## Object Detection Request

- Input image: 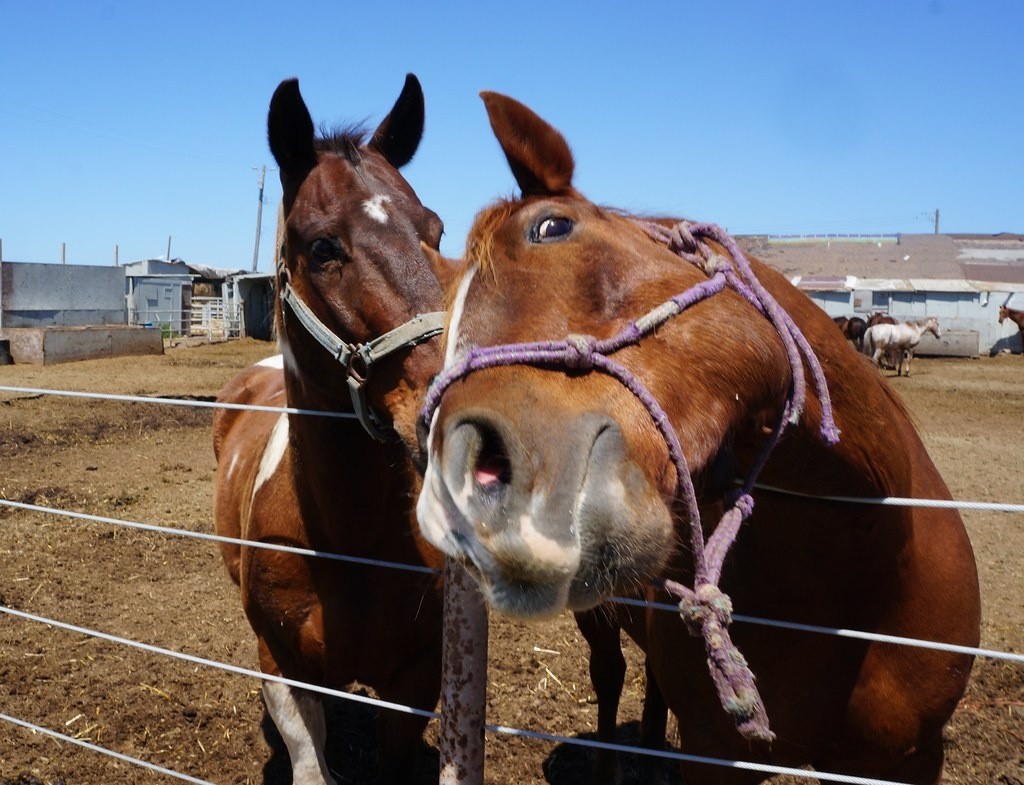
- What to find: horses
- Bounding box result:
[214,72,1023,785]
